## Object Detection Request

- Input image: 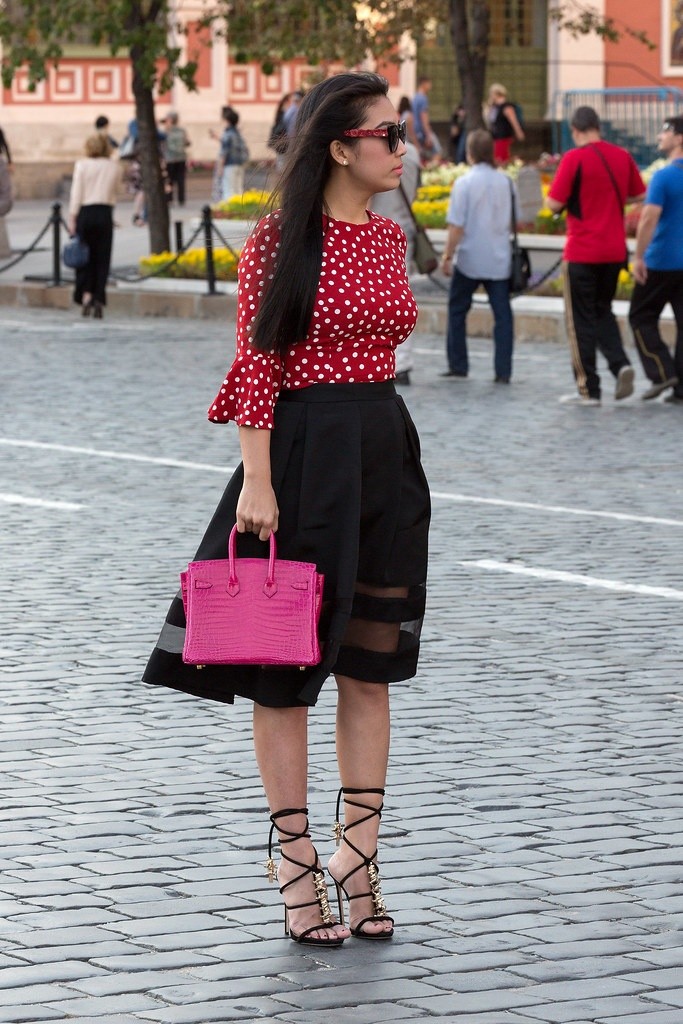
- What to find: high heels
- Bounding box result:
[329,783,395,940]
[267,807,351,946]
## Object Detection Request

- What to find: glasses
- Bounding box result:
[344,121,406,154]
[663,122,677,134]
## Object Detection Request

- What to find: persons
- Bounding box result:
[366,139,421,387]
[128,110,190,227]
[95,116,121,229]
[397,76,443,168]
[548,106,646,406]
[209,106,250,204]
[436,129,521,386]
[0,126,16,217]
[69,132,116,319]
[628,115,683,404]
[448,83,527,167]
[274,91,306,179]
[141,71,431,945]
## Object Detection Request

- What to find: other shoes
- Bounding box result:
[665,396,683,405]
[81,299,102,320]
[441,371,468,383]
[643,378,678,399]
[615,365,635,400]
[393,371,408,386]
[561,392,601,406]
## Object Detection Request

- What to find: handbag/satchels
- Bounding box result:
[62,233,89,268]
[506,245,531,292]
[180,520,323,671]
[413,229,437,273]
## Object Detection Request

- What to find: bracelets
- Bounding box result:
[442,254,453,260]
